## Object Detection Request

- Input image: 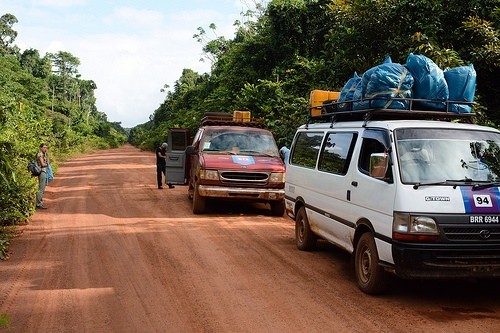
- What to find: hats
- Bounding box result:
[161,142,167,148]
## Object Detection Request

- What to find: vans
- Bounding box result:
[285,97,500,294]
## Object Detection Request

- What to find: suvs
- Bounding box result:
[165,116,286,216]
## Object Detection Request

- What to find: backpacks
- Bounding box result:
[46,163,54,186]
[28,153,41,175]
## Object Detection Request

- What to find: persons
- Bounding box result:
[36,143,49,209]
[155,143,175,189]
[278,143,290,166]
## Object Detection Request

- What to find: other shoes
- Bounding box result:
[38,206,47,209]
[157,186,162,188]
[169,185,175,188]
